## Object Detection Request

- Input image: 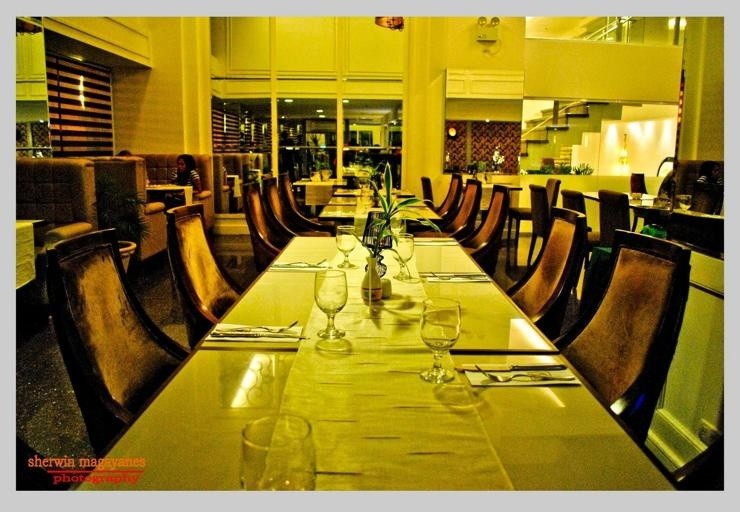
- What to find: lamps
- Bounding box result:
[476,15,499,42]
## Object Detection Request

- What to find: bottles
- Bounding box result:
[446,153,451,169]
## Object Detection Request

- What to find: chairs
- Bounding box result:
[15,144,722,490]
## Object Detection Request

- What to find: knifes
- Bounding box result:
[420,273,486,277]
[455,365,567,371]
[272,265,332,269]
[211,331,312,339]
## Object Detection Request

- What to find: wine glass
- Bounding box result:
[314,270,348,338]
[392,232,414,281]
[336,225,357,268]
[420,298,460,384]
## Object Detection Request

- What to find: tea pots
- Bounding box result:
[310,172,321,181]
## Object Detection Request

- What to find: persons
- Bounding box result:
[280,130,401,183]
[164,154,202,226]
[222,167,227,184]
[694,161,723,214]
[118,151,150,187]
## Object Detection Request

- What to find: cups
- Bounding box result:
[357,179,372,217]
[677,194,692,211]
[237,414,318,490]
[347,161,362,175]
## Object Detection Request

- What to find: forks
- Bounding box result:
[276,259,325,266]
[430,271,489,282]
[217,322,297,333]
[473,362,576,381]
